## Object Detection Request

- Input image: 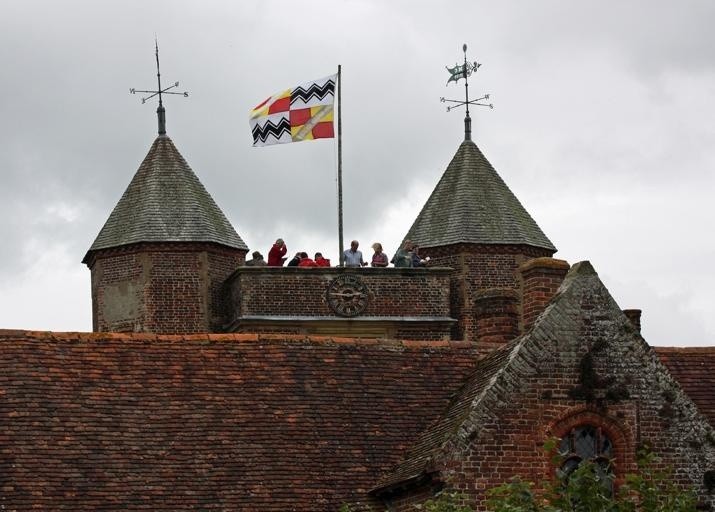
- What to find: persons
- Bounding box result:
[396,240,413,267]
[413,245,428,267]
[371,243,388,267]
[244,237,331,267]
[343,240,368,267]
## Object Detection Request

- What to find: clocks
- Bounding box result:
[327,276,369,318]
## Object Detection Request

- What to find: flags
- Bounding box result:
[248,72,338,147]
